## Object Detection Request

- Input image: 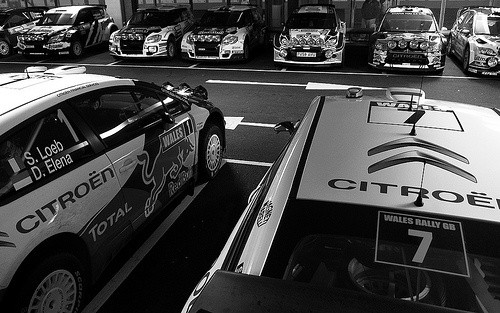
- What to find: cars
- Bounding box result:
[366,4,450,78]
[178,86,500,313]
[0,63,227,313]
[12,3,119,60]
[272,3,348,69]
[446,5,500,78]
[0,5,52,58]
[107,4,201,62]
[179,3,270,66]
